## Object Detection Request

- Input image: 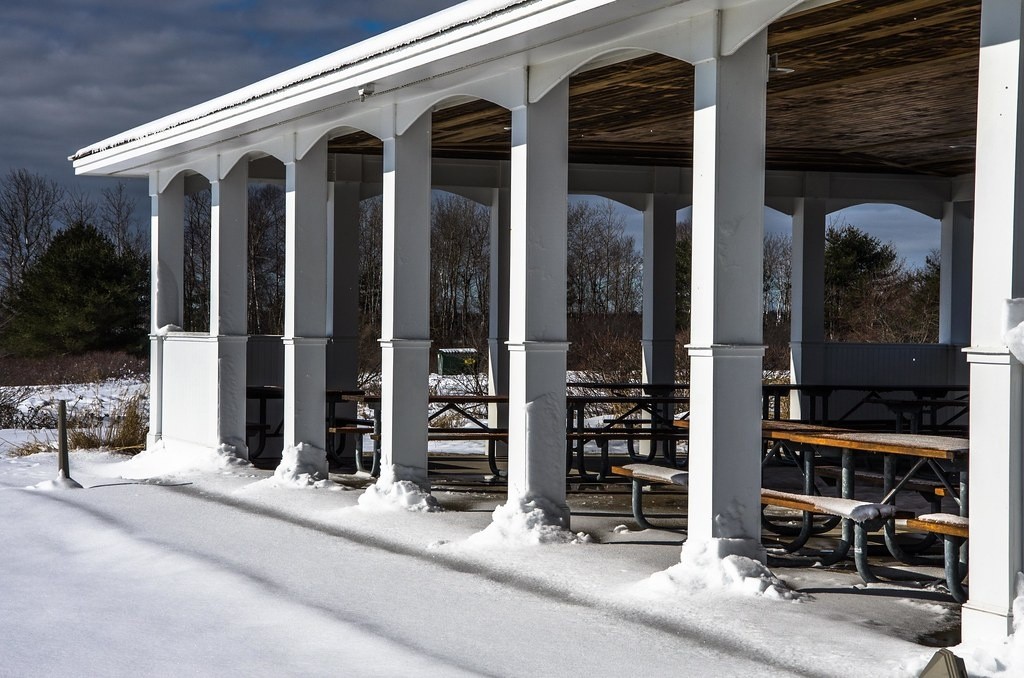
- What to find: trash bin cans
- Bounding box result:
[436,348,479,375]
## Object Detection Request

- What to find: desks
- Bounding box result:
[565,382,790,481]
[246,386,365,468]
[341,394,509,477]
[672,418,970,603]
[773,384,969,439]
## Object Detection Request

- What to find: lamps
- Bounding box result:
[767,52,795,83]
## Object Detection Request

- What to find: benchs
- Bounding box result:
[326,416,374,459]
[565,398,969,481]
[246,422,270,459]
[611,465,970,603]
[329,427,509,477]
